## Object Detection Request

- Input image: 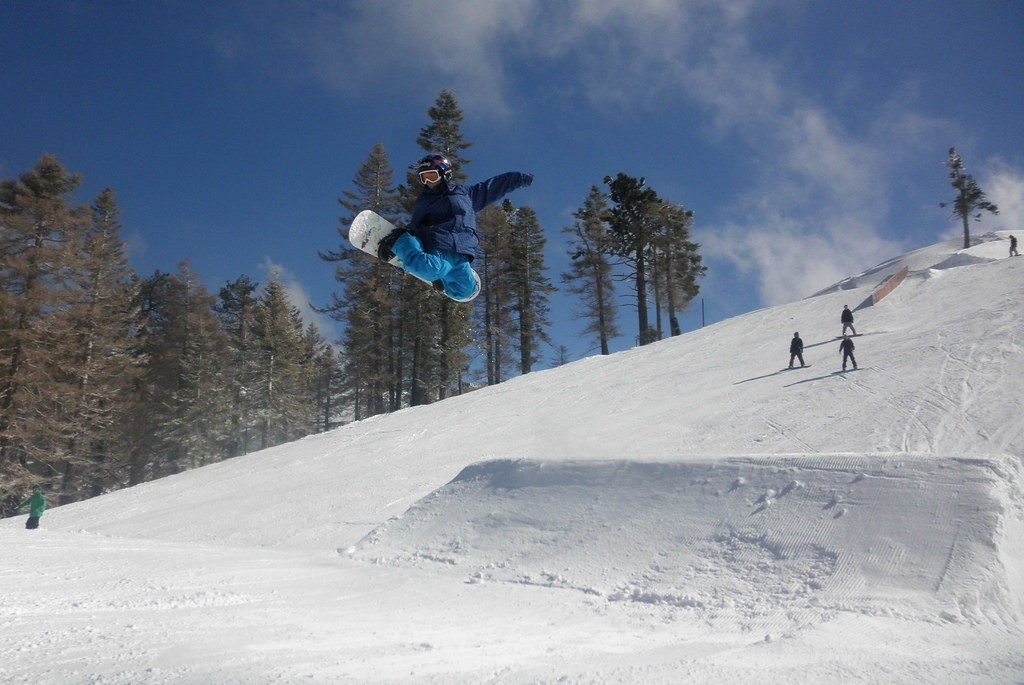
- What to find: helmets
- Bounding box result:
[416,154,452,182]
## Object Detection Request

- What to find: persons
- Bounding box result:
[14,484,45,529]
[841,305,856,336]
[378,155,534,303]
[1009,235,1018,256]
[789,332,805,367]
[839,333,858,371]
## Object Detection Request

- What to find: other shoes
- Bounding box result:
[432,280,444,292]
[378,229,409,264]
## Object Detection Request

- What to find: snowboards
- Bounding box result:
[348,209,482,303]
[778,364,813,373]
[830,367,865,375]
[834,333,864,340]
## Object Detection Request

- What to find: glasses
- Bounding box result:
[418,170,442,185]
